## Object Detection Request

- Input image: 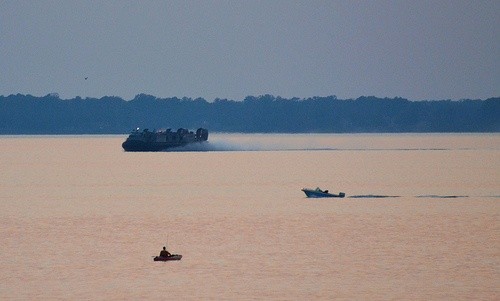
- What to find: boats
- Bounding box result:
[302,188,345,198]
[122,127,208,153]
[154,254,181,262]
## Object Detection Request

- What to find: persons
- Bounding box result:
[160,247,171,257]
